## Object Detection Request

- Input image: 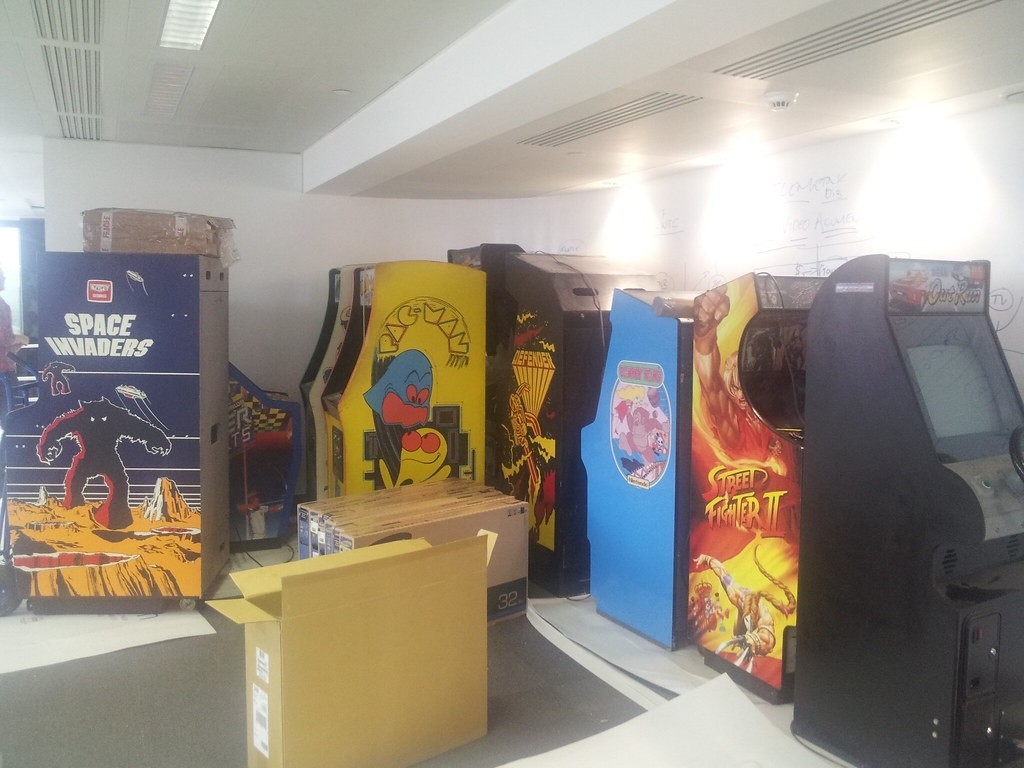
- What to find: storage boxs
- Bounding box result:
[296,476,531,630]
[201,528,499,767]
[83,207,238,256]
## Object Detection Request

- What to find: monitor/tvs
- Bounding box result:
[906,344,1003,437]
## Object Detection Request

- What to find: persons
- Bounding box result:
[0,264,29,432]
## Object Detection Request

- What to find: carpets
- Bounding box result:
[0,595,648,768]
